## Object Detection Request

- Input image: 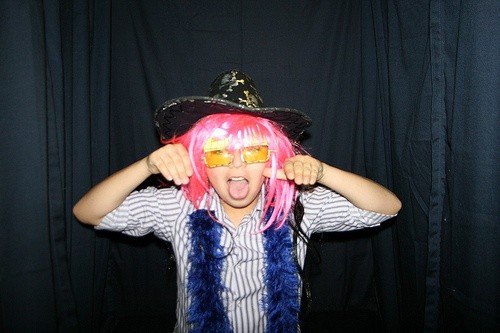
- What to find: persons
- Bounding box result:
[72,71,402,333]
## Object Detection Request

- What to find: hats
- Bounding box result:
[154,69,311,145]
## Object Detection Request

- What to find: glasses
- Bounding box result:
[203,144,270,168]
